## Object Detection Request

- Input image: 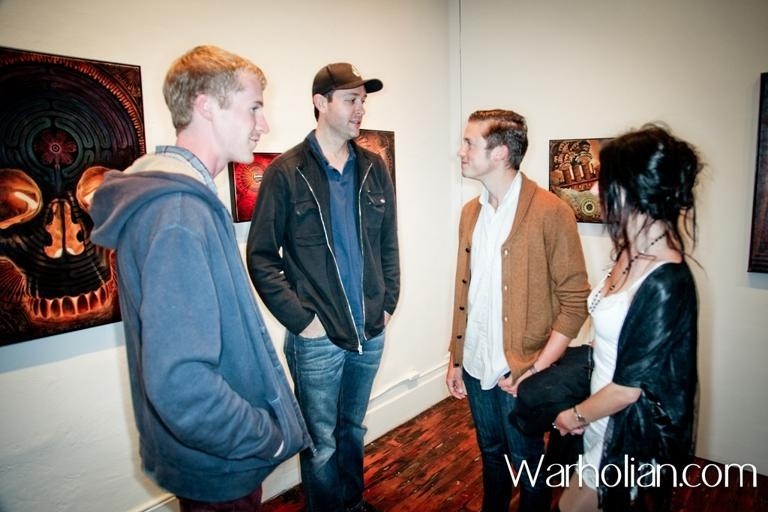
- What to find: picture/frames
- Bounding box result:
[355,128,397,197]
[745,71,768,275]
[0,43,148,350]
[226,151,283,224]
[547,136,611,225]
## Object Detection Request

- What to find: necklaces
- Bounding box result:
[588,230,666,315]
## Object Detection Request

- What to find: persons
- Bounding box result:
[246,62,400,512]
[550,123,703,512]
[444,110,592,512]
[88,45,317,512]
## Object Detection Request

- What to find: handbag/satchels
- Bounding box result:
[509,344,594,440]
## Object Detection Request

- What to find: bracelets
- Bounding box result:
[572,405,590,429]
[529,366,539,375]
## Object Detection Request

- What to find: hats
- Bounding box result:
[313,62,384,97]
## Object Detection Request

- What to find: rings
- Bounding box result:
[551,421,557,429]
